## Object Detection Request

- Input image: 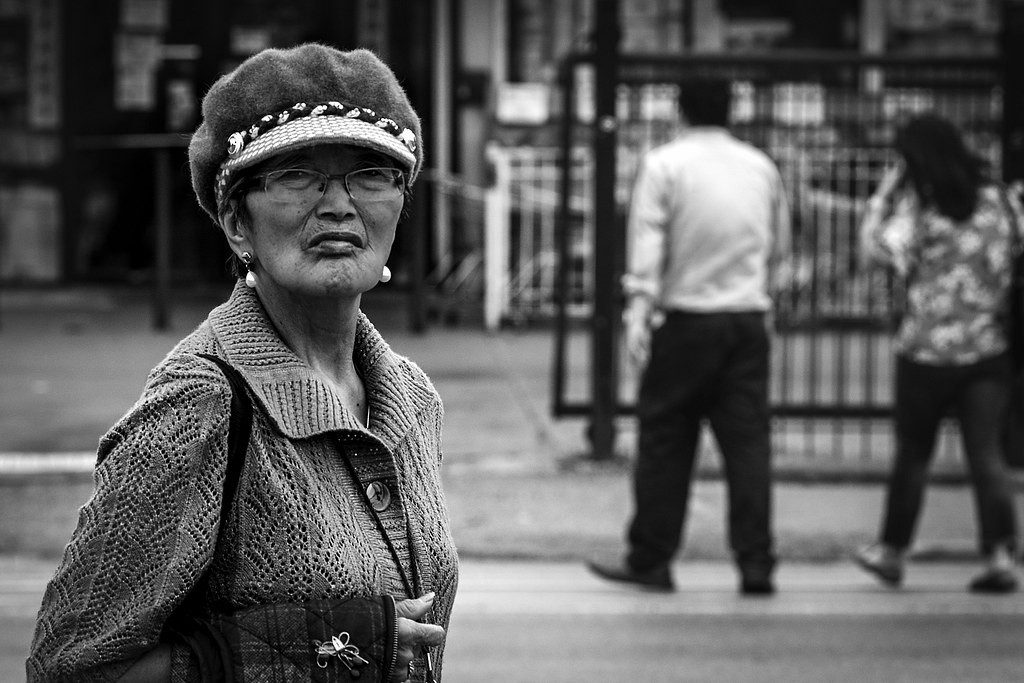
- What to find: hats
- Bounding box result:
[188,44,423,228]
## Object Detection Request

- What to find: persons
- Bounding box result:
[852,113,1023,593]
[24,43,459,683]
[588,67,792,596]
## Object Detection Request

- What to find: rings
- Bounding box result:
[408,662,414,679]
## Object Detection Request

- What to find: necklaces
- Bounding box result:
[354,363,370,427]
[338,450,436,683]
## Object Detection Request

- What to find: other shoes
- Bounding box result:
[588,553,671,589]
[967,565,1019,593]
[853,544,905,587]
[740,575,776,595]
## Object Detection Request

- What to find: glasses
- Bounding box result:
[248,169,410,204]
